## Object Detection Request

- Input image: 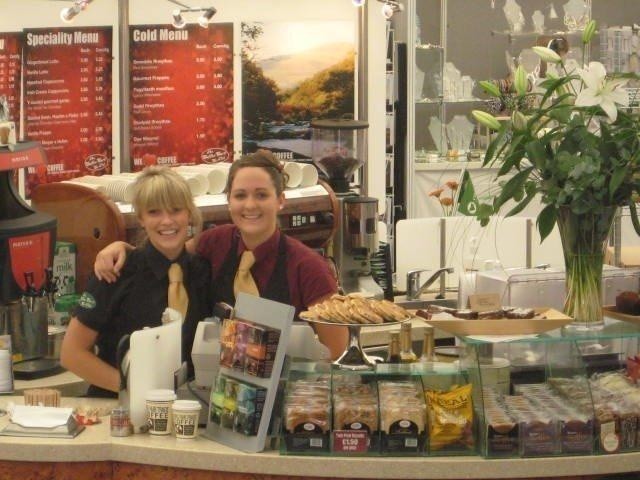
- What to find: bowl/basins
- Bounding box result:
[406,305,575,336]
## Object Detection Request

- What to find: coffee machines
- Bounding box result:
[311,117,388,302]
[29,179,345,297]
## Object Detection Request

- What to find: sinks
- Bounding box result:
[395,299,459,309]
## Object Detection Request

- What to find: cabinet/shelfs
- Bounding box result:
[405,0,640,164]
[405,164,640,220]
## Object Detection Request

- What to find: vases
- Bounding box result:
[553,204,622,326]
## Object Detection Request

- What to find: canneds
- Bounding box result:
[110,409,131,437]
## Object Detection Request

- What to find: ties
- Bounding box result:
[232,248,262,304]
[165,261,190,328]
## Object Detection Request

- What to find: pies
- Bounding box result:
[300,299,405,324]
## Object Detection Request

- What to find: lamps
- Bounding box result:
[353,1,405,19]
[59,0,93,22]
[167,0,217,28]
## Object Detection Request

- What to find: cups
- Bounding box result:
[65,160,325,206]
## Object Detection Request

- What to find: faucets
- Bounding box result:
[406,267,454,302]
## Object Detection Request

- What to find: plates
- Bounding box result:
[601,303,640,325]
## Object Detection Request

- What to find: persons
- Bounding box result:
[94,150,339,323]
[64,167,214,396]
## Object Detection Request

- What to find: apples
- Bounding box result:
[616,292,638,312]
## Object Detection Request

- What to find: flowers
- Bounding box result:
[470,13,640,243]
[428,176,462,215]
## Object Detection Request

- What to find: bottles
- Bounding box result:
[386,321,439,364]
[109,387,201,440]
[0,333,15,394]
